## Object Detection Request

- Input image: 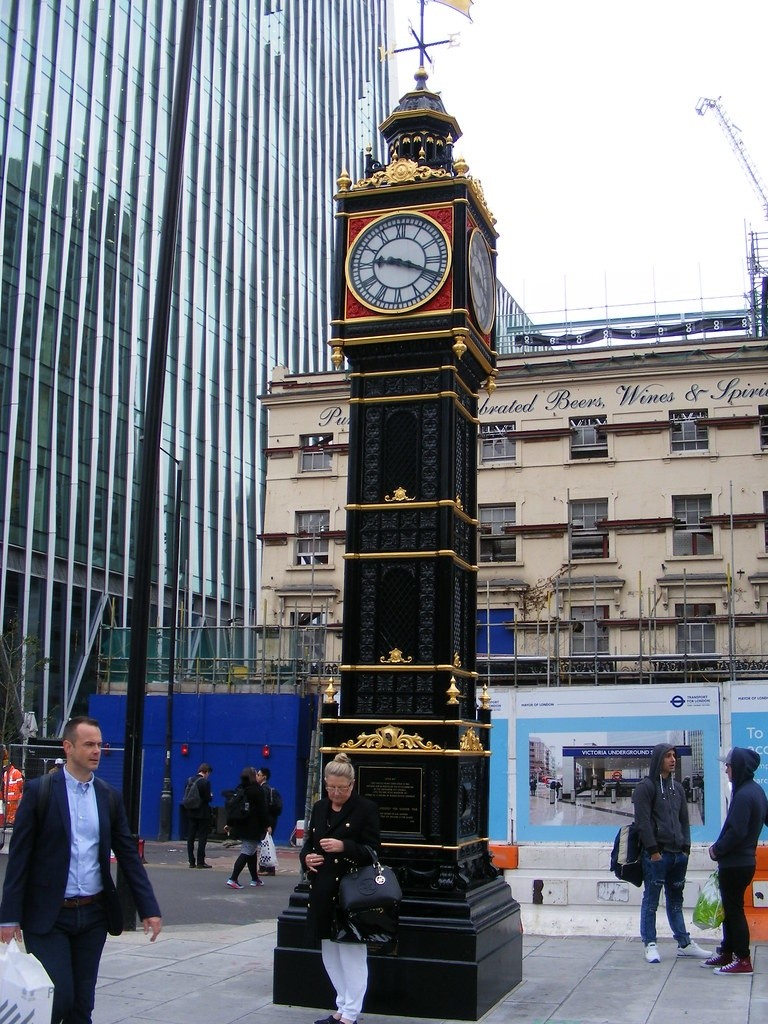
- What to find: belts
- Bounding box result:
[63,893,101,907]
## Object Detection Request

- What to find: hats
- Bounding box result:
[716,747,734,765]
[54,758,63,765]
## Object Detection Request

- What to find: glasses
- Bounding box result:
[325,781,352,794]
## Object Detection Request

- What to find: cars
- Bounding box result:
[541,776,557,783]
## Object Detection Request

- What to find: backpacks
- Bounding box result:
[265,786,283,816]
[183,776,205,808]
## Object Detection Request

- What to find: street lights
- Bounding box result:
[139,436,182,839]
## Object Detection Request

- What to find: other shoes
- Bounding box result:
[313,1015,357,1024]
[249,879,264,886]
[227,880,245,889]
[197,863,213,868]
[189,863,197,868]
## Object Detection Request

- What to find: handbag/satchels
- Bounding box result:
[340,845,402,908]
[610,824,645,888]
[693,868,725,930]
[0,933,54,1024]
[258,832,278,867]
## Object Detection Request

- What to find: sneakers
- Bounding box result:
[643,942,661,962]
[713,956,754,975]
[676,939,713,958]
[699,953,735,967]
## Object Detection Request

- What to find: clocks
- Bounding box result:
[467,228,496,334]
[348,214,454,317]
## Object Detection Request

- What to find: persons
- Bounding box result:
[182,763,212,869]
[3,759,24,828]
[681,774,704,803]
[256,767,277,876]
[631,743,711,962]
[530,779,562,799]
[49,757,66,775]
[699,747,768,975]
[223,767,273,889]
[298,752,381,1024]
[0,715,164,1024]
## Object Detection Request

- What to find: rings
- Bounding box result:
[311,858,312,863]
